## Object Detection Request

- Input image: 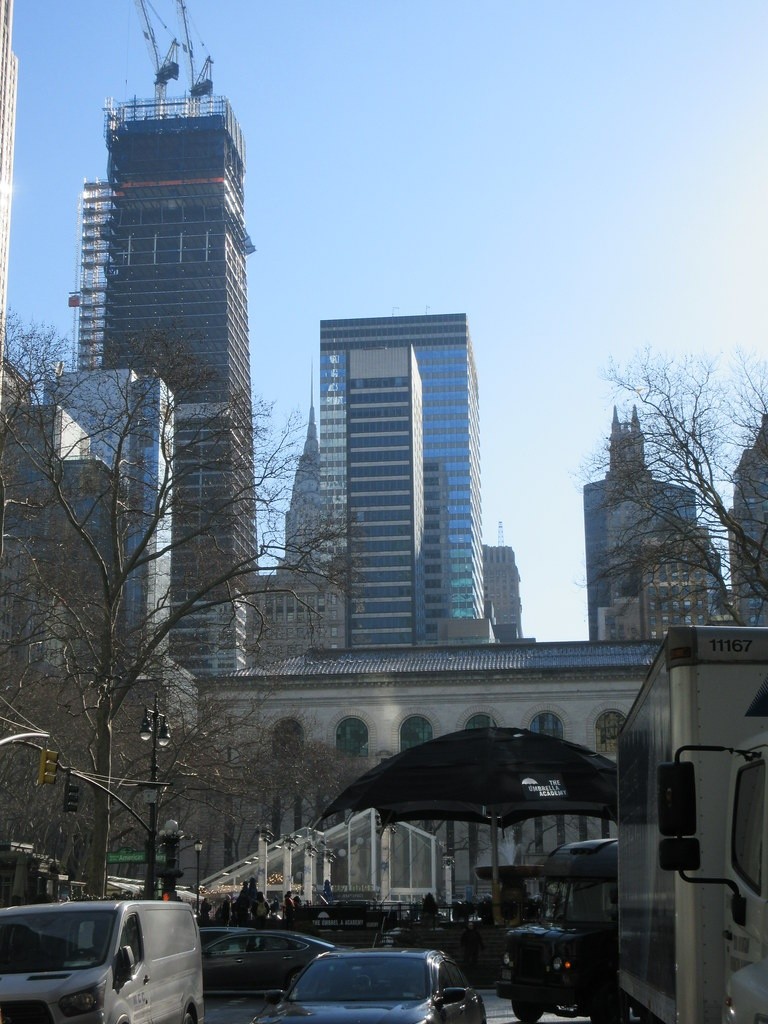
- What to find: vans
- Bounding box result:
[0,898,205,1024]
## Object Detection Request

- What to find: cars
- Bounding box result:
[201,929,364,996]
[248,946,489,1024]
[199,926,257,949]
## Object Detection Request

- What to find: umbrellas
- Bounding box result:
[308,728,617,926]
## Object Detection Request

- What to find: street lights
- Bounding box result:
[326,849,336,881]
[284,835,302,892]
[138,687,172,901]
[222,872,241,893]
[156,819,186,902]
[387,826,397,901]
[306,843,319,904]
[260,826,275,900]
[194,838,204,918]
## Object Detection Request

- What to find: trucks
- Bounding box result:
[611,622,768,1024]
[493,836,630,1024]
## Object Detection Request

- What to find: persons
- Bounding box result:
[201,878,301,930]
[460,922,484,967]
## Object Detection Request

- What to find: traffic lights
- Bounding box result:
[61,781,80,813]
[36,744,59,786]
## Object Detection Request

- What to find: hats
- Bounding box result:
[225,894,231,900]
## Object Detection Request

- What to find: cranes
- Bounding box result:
[138,0,217,100]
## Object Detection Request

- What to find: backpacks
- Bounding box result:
[255,900,267,916]
